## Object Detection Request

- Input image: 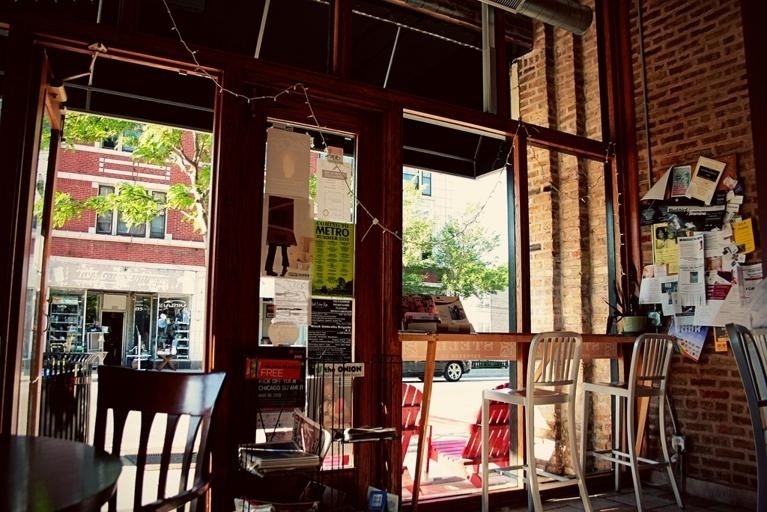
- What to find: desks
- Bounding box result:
[0,434,122,510]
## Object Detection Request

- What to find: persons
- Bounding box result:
[655,227,667,249]
[664,227,677,248]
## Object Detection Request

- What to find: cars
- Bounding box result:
[401,360,474,385]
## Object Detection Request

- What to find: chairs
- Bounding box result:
[724,322,767,511]
[92,365,226,511]
[481,332,685,512]
